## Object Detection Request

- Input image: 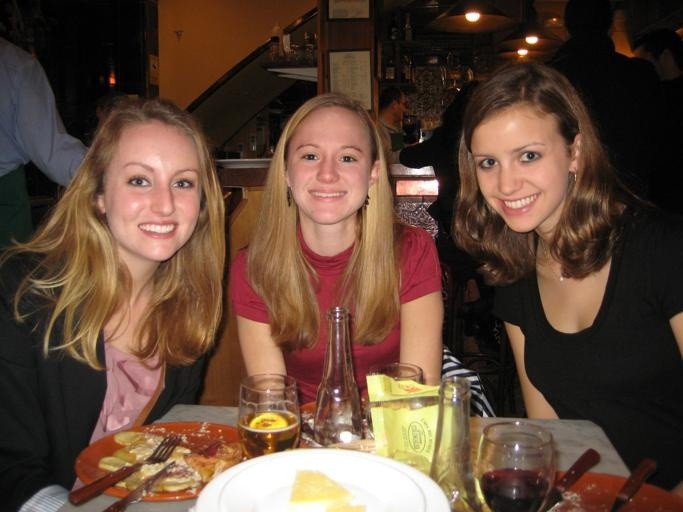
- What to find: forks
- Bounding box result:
[68,433,182,512]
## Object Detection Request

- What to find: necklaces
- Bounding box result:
[539,239,566,282]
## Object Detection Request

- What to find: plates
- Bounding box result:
[195,447,449,511]
[74,422,249,502]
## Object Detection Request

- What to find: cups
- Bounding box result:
[476,420,553,512]
[238,373,302,459]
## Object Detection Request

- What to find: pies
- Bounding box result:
[97,437,244,491]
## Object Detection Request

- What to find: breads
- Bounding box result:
[291,470,368,511]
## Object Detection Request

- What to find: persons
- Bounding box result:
[453,59,683,501]
[0,35,91,271]
[377,84,411,162]
[0,97,226,512]
[66,90,129,148]
[628,25,683,214]
[230,92,445,398]
[400,79,500,365]
[550,1,660,202]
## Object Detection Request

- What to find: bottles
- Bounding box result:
[312,305,366,450]
[430,378,482,511]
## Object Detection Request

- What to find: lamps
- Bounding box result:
[428,2,564,61]
